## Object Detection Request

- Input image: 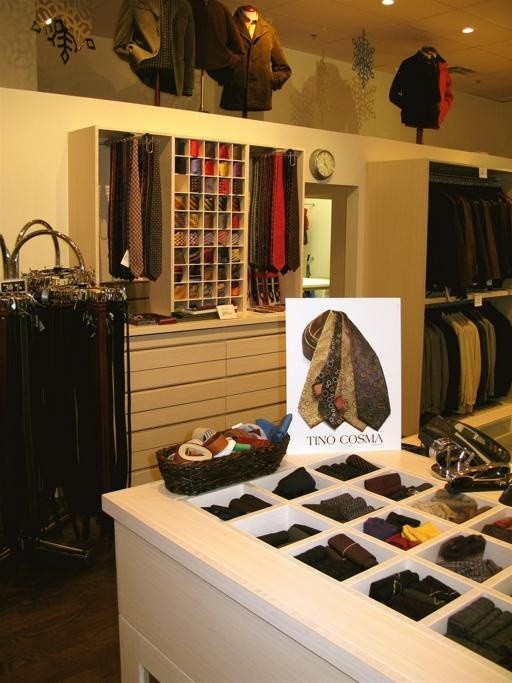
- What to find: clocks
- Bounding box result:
[311,149,337,177]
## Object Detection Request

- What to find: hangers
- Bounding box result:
[428,170,504,195]
[427,299,498,325]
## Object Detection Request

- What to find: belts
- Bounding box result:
[0,284,136,555]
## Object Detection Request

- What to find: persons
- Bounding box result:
[184,0,248,72]
[204,5,294,111]
[112,0,196,98]
[388,46,456,129]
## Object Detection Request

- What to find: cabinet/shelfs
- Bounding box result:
[363,156,509,462]
[68,126,305,490]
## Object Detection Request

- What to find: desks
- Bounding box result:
[98,437,511,681]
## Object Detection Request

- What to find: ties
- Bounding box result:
[105,132,301,329]
[297,309,394,432]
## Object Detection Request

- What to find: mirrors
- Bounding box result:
[301,193,345,300]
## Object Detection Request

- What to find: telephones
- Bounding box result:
[418,413,511,476]
